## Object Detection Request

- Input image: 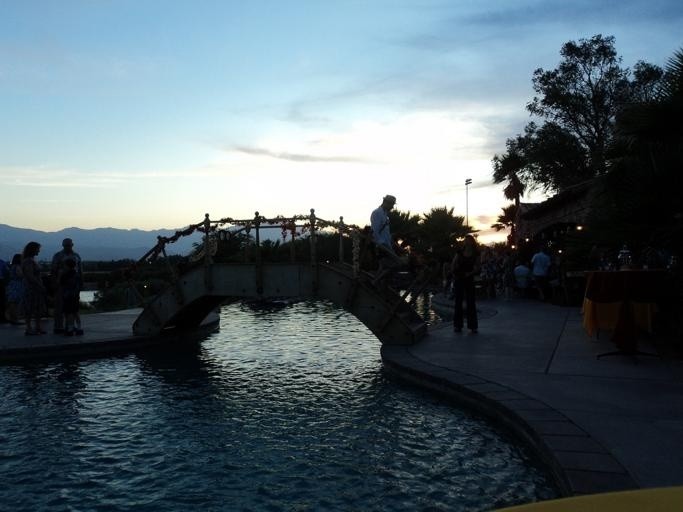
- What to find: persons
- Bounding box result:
[450,235,482,333]
[51,238,83,337]
[0,242,48,336]
[443,244,550,300]
[370,195,396,253]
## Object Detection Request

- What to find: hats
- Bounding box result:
[63,256,74,269]
[383,195,396,204]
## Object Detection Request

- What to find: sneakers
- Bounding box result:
[54,328,65,334]
[78,329,84,336]
[24,328,48,336]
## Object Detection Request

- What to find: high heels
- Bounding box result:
[454,327,463,332]
[470,327,478,333]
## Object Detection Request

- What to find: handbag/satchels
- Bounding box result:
[373,246,390,260]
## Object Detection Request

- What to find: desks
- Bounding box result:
[582,269,683,366]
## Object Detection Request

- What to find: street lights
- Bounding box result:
[466,178,472,225]
